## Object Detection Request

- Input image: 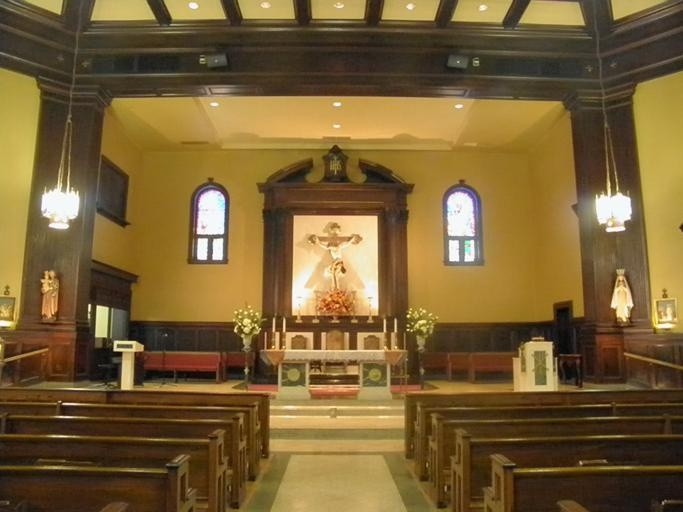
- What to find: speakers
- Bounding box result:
[446,54,470,68]
[206,54,228,68]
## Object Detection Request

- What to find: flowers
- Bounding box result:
[406,307,440,338]
[233,305,262,338]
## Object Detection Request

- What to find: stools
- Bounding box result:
[556,354,583,388]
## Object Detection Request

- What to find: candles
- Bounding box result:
[394,318,397,332]
[383,319,386,331]
[283,317,286,332]
[272,318,276,332]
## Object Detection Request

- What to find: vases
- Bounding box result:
[241,334,253,390]
[416,334,426,390]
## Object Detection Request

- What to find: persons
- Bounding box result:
[310,223,357,291]
[41,271,60,320]
[40,270,51,293]
[610,276,635,323]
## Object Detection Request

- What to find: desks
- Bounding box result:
[259,349,408,391]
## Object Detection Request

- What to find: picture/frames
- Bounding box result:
[654,298,677,324]
[0,297,16,321]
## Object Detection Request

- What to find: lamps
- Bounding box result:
[41,0,86,229]
[592,0,632,232]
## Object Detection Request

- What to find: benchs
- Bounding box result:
[145,351,255,383]
[404,389,683,512]
[0,386,269,512]
[425,352,560,381]
